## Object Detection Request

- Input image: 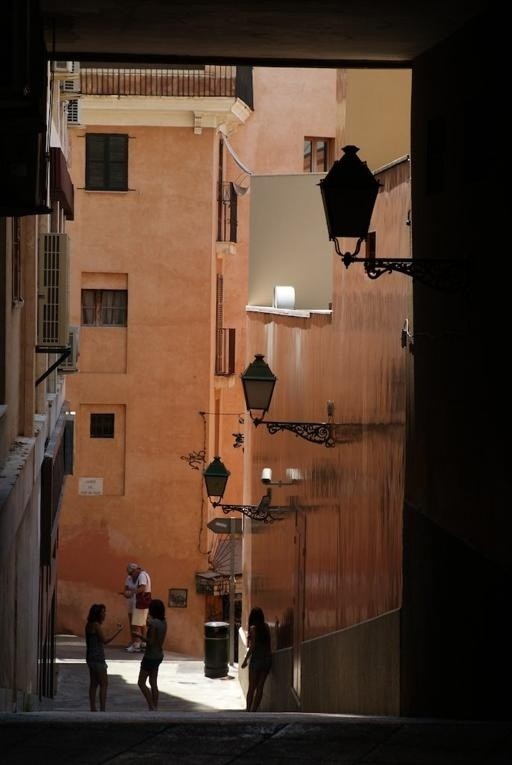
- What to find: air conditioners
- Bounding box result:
[56,328,80,368]
[54,59,81,125]
[39,234,71,347]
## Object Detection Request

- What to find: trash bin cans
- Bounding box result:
[204,621,230,678]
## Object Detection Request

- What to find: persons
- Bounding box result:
[133,600,167,711]
[242,607,270,712]
[119,575,137,649]
[85,604,124,711]
[125,563,151,652]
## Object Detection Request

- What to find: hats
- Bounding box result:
[127,563,138,575]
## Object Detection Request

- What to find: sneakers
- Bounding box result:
[140,641,146,651]
[124,643,141,652]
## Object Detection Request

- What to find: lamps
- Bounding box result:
[203,456,270,521]
[239,354,334,449]
[316,146,414,281]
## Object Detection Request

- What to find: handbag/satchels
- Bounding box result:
[135,573,152,609]
